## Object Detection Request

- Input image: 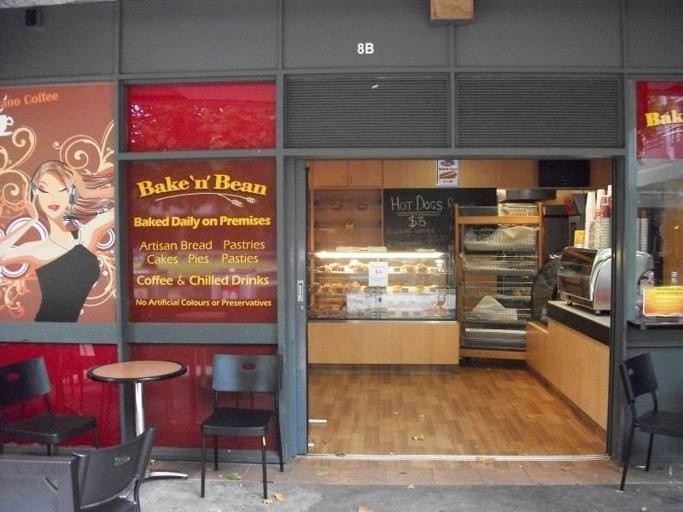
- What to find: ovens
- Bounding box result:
[557,246,654,315]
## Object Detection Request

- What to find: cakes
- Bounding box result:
[318,262,437,294]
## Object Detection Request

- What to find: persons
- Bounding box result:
[0,156,117,323]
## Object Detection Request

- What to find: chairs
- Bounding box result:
[620,352,682,491]
[1,356,97,456]
[69,425,155,512]
[201,352,283,500]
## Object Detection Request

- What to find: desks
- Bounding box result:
[87,359,189,481]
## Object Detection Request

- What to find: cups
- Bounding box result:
[574,184,648,253]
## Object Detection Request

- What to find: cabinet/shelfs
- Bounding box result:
[311,187,386,250]
[306,252,461,365]
[456,204,545,361]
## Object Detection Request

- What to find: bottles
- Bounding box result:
[671,272,678,286]
